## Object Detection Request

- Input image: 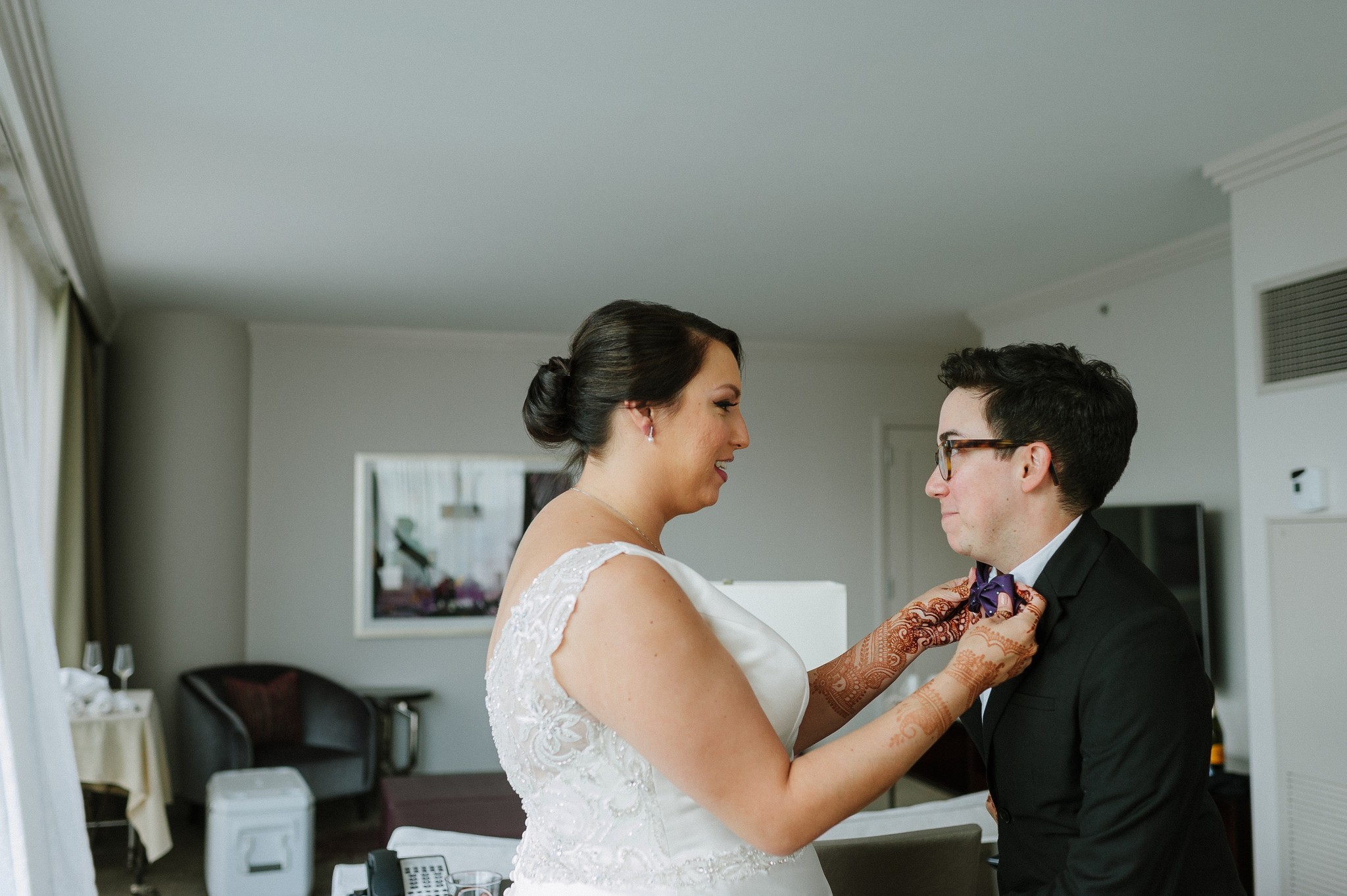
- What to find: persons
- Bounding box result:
[926,344,1215,896]
[485,299,1046,896]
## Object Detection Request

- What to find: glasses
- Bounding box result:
[935,439,1061,487]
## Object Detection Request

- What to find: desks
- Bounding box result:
[351,689,431,774]
[70,688,174,896]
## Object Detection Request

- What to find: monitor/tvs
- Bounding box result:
[1088,503,1213,682]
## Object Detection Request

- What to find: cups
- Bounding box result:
[82,641,104,674]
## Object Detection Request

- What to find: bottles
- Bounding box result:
[1208,704,1225,777]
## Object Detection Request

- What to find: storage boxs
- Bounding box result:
[204,766,317,896]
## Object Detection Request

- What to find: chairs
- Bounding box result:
[382,773,526,844]
[177,663,380,830]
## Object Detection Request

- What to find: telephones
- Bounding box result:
[365,850,458,896]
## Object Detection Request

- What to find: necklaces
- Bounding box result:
[569,487,664,555]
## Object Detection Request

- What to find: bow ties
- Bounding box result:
[965,560,1022,619]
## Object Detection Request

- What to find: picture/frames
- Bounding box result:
[354,452,581,639]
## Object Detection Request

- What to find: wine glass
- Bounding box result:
[113,644,134,691]
